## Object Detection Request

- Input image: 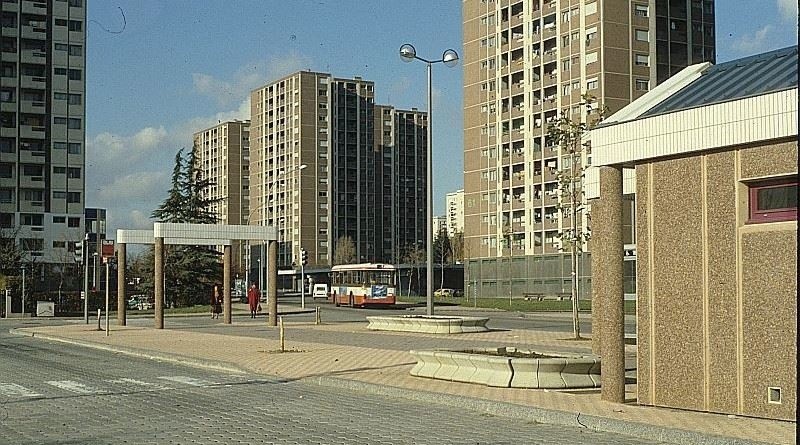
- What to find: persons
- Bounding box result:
[248,283,261,319]
[210,285,223,320]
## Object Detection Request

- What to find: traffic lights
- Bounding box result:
[73,239,84,266]
[300,247,309,267]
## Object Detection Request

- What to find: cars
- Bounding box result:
[127,293,169,311]
[312,283,329,300]
[433,288,464,297]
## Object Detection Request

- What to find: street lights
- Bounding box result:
[245,199,276,298]
[400,43,459,316]
[267,165,307,303]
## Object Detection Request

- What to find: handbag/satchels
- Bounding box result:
[257,304,262,311]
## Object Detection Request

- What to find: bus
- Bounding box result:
[330,263,398,309]
